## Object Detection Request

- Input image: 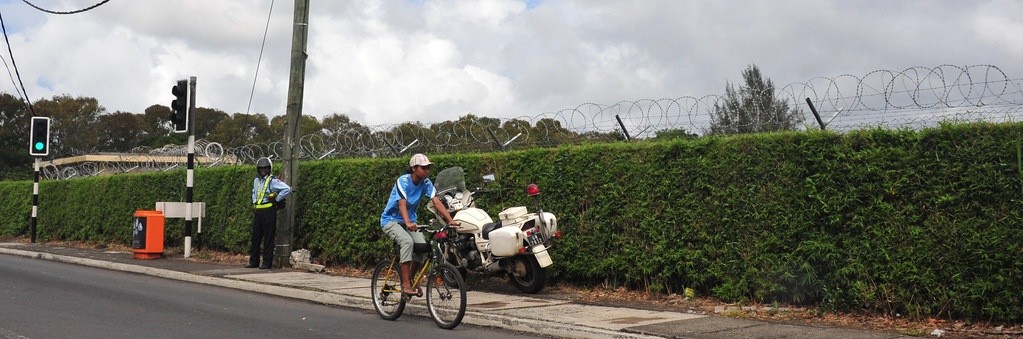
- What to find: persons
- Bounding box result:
[244,157,291,269]
[380,153,458,296]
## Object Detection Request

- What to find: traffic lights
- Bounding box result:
[170,80,190,133]
[29,116,50,157]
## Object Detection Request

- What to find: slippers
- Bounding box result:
[398,287,417,294]
[411,279,423,297]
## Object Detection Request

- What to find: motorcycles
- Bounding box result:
[426,166,562,294]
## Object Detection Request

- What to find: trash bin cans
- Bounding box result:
[133,210,165,259]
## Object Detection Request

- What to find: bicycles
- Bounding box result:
[371,218,467,330]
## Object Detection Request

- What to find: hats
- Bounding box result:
[409,154,433,167]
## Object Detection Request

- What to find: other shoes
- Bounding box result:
[244,264,259,268]
[259,265,271,269]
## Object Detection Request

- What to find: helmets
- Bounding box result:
[256,157,272,167]
